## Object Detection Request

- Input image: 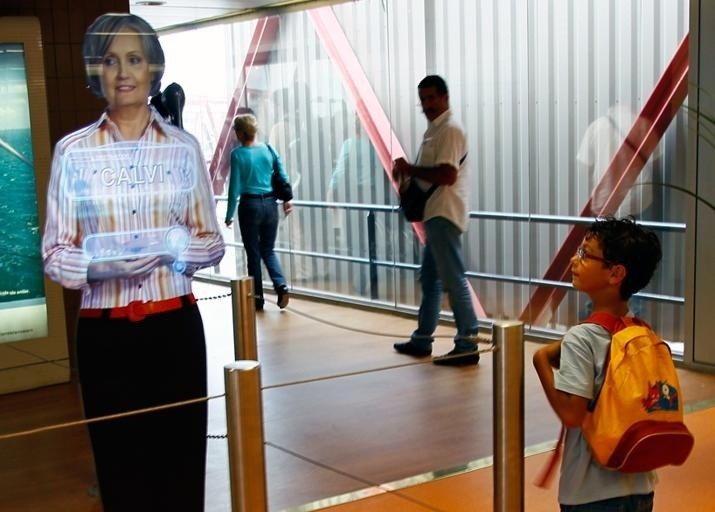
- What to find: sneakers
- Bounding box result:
[432,342,480,367]
[394,336,433,358]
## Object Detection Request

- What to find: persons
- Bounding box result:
[532,216,662,511]
[578,98,660,322]
[392,75,480,367]
[328,117,388,296]
[42,13,225,511]
[225,113,292,313]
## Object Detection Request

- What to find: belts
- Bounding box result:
[76,293,198,324]
[241,191,276,200]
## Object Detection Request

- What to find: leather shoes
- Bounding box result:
[279,285,289,308]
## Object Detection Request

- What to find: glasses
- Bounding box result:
[576,246,617,266]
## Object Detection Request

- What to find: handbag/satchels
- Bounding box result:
[395,175,428,224]
[272,171,293,203]
[572,325,695,477]
